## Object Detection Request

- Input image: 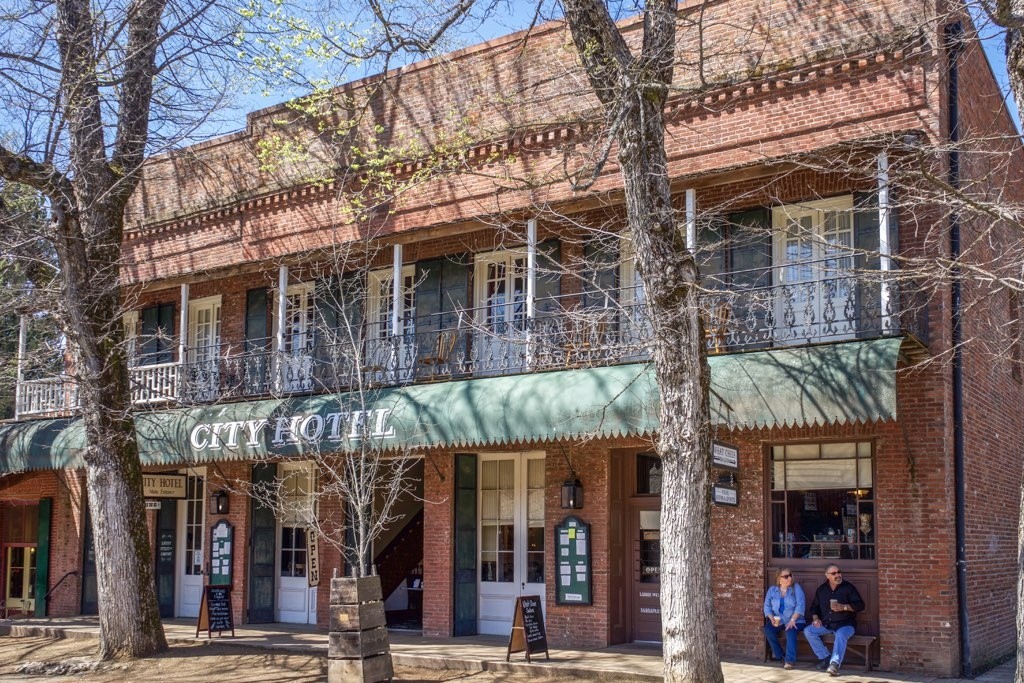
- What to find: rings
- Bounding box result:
[815,622,817,623]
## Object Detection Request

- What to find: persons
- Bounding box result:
[763,567,806,669]
[803,563,865,675]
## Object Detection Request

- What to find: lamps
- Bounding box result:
[561,469,584,511]
[209,488,230,515]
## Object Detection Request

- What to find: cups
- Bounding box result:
[774,616,781,627]
[830,599,837,610]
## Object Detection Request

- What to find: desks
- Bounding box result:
[400,587,423,623]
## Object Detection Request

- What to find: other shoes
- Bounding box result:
[777,659,784,666]
[784,662,794,670]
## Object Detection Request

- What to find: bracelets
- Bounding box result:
[791,619,794,621]
[844,604,847,610]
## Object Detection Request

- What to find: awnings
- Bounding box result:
[0,337,911,473]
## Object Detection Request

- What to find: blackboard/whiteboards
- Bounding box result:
[197,585,234,631]
[508,595,548,654]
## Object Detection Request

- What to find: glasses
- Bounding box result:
[781,573,792,579]
[828,571,841,575]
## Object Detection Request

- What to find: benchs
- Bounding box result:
[778,629,876,672]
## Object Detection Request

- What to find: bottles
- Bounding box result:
[837,530,841,535]
[411,566,423,574]
[828,527,835,535]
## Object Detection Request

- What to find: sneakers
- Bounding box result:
[827,661,839,675]
[816,661,828,670]
